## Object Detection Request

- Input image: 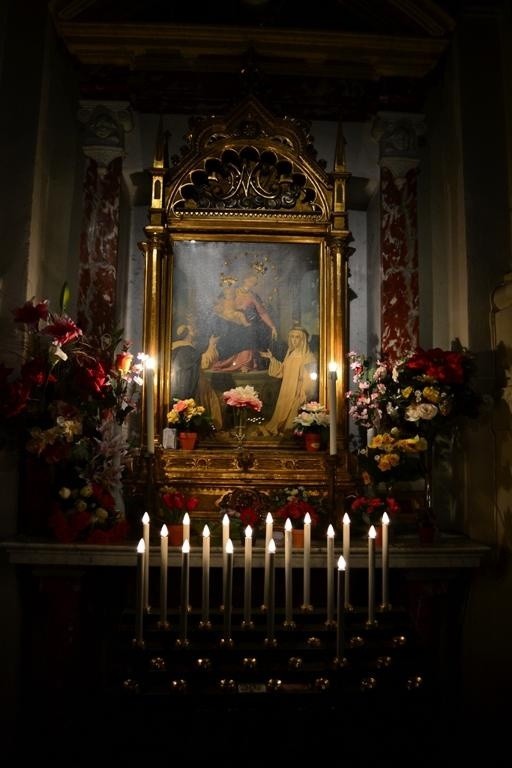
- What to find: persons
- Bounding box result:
[171,324,223,432]
[207,287,251,329]
[235,271,278,341]
[258,326,318,436]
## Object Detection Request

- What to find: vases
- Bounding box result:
[233,425,249,469]
[305,434,322,451]
[179,432,199,451]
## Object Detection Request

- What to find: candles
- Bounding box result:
[145,375,156,453]
[329,373,337,454]
[135,512,392,665]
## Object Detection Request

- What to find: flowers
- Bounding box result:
[6,295,133,542]
[158,489,400,522]
[220,384,263,427]
[343,343,475,475]
[166,398,206,432]
[294,399,329,435]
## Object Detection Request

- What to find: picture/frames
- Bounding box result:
[168,231,325,449]
